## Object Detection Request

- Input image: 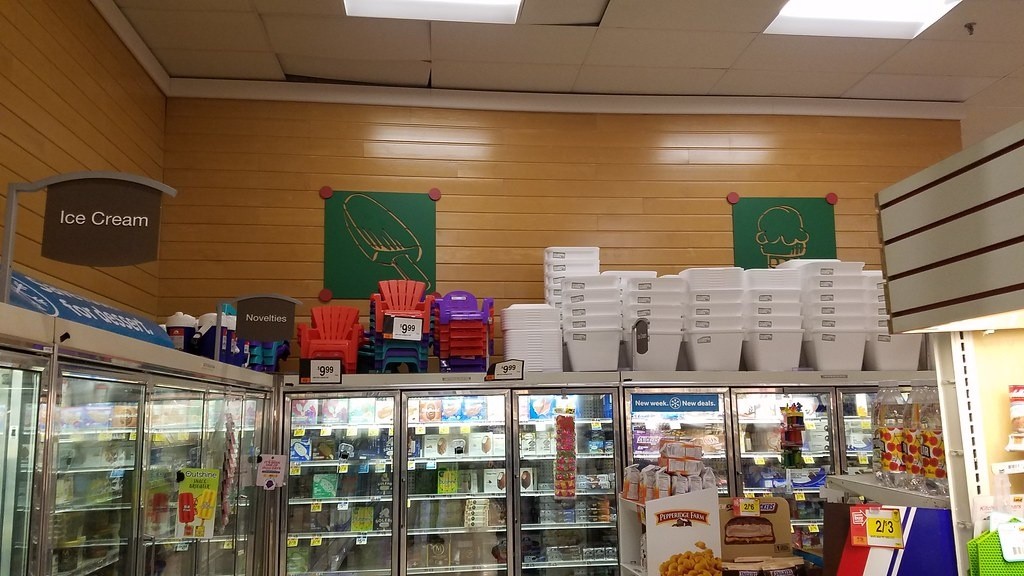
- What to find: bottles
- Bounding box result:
[872,379,949,497]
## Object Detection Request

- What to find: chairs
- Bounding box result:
[221,280,495,374]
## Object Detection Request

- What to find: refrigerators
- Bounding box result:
[0,302,938,576]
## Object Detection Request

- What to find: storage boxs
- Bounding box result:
[543,246,923,371]
[292,395,873,563]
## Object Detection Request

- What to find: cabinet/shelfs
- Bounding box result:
[1,347,279,576]
[275,372,941,576]
[619,486,723,576]
